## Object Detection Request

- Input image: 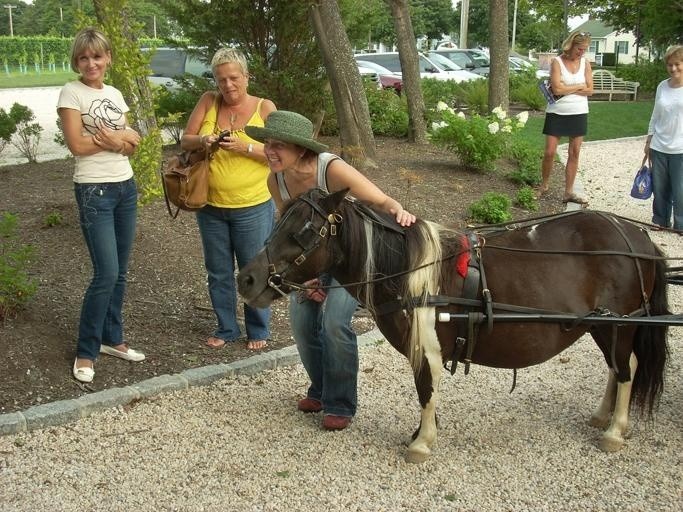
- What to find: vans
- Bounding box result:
[137,43,278,104]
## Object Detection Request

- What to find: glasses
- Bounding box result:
[578,31,591,36]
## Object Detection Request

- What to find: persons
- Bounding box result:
[56,27,147,383]
[180,47,278,350]
[537,30,593,204]
[643,44,683,234]
[245,110,417,430]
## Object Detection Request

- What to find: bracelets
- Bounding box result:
[247,143,253,156]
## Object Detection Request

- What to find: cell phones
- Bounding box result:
[216,130,230,146]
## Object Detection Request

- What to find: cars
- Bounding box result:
[356,60,403,98]
[479,45,533,72]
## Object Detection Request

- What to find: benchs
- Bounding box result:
[591,69,640,102]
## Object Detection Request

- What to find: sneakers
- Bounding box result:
[298,397,322,412]
[322,414,349,429]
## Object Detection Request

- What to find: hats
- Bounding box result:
[244,110,330,153]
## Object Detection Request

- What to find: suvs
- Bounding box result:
[356,67,383,92]
[430,49,490,77]
[353,53,486,84]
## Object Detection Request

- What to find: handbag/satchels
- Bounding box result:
[159,146,209,219]
[630,152,652,199]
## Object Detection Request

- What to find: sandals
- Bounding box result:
[73,357,95,383]
[532,186,548,200]
[562,192,588,204]
[100,345,145,362]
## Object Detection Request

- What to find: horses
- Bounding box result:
[236,187,668,464]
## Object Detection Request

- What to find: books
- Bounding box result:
[538,76,566,104]
